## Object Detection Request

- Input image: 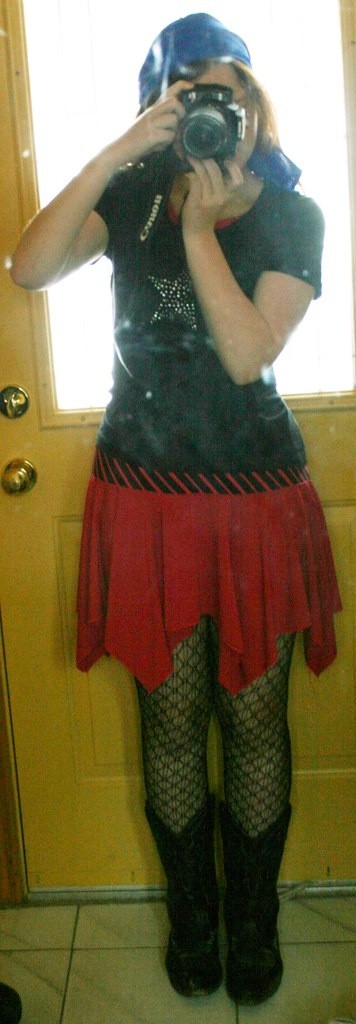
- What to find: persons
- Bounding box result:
[9,12,344,1005]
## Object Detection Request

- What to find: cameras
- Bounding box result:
[174,83,246,176]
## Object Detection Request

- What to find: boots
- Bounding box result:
[143,795,223,996]
[218,798,292,1006]
